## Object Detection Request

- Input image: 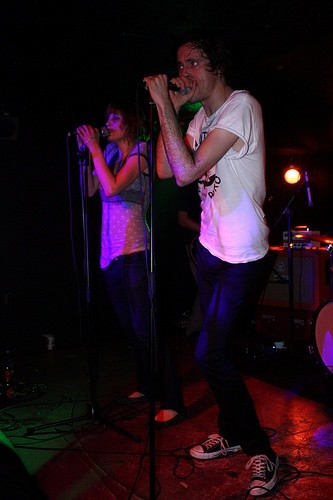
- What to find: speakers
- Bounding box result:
[257,246,333,311]
[0,431,36,500]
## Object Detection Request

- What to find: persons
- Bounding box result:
[76,101,186,427]
[141,39,282,496]
[177,190,214,326]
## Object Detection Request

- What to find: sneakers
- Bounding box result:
[245,448,279,496]
[189,433,243,459]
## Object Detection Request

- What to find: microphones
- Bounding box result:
[167,81,192,95]
[278,279,289,284]
[68,127,110,137]
[305,171,313,207]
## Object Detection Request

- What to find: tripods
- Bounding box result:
[27,135,141,443]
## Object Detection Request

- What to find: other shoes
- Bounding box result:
[121,391,150,406]
[154,409,185,429]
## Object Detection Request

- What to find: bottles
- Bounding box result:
[0,349,48,400]
